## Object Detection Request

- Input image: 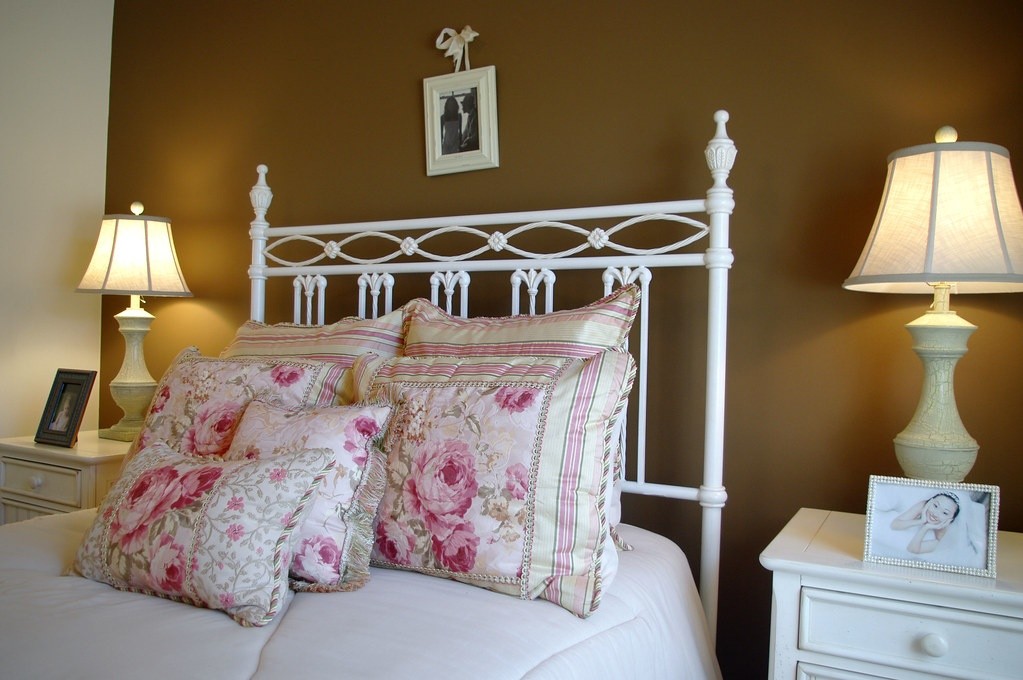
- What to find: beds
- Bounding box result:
[0,108,737,680]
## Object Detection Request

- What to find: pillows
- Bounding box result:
[60,438,337,627]
[115,347,357,465]
[221,306,406,364]
[349,351,636,619]
[223,400,395,592]
[404,280,643,354]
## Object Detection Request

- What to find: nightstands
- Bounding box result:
[757,507,1023,680]
[0,431,132,524]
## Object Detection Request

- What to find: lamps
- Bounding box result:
[841,125,1023,483]
[74,200,194,443]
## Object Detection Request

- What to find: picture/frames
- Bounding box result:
[34,368,97,448]
[423,65,500,177]
[863,474,1000,579]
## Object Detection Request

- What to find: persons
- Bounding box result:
[871,484,987,570]
[459,94,478,152]
[48,392,72,432]
[440,96,462,155]
